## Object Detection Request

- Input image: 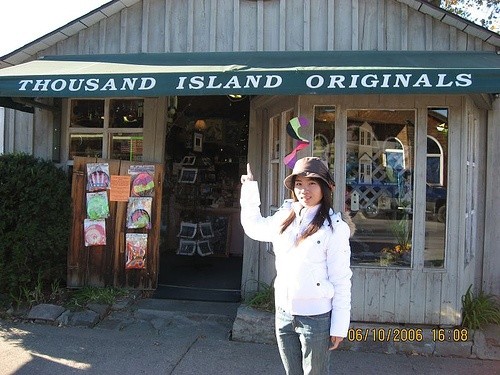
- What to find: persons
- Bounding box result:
[240,157,353,375]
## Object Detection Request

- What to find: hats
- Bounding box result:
[284,156,336,189]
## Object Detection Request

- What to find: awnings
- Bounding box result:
[0,51,500,113]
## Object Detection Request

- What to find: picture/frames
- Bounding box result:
[193,133,204,154]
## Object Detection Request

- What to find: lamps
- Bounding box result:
[194,120,208,132]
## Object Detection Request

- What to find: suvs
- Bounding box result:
[346,162,447,223]
